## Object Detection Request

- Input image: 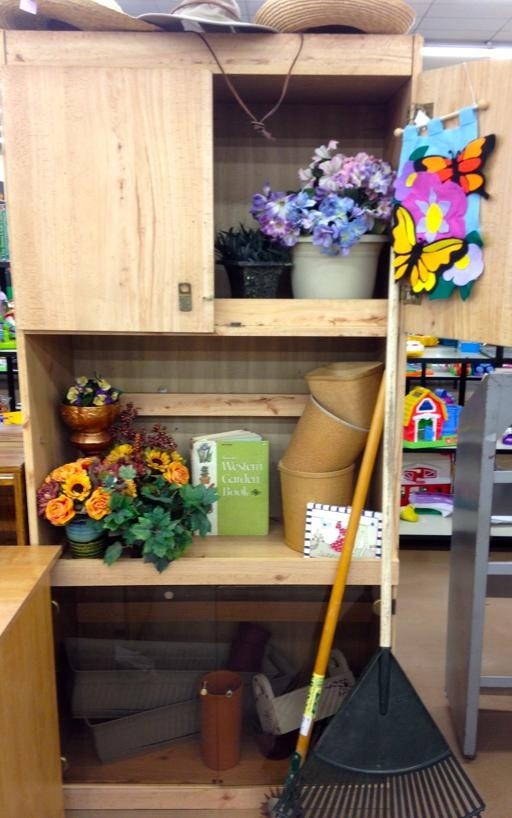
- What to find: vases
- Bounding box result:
[292,236,385,299]
[58,405,122,447]
[63,519,106,558]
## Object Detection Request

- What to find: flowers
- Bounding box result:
[250,141,393,257]
[62,377,121,405]
[39,432,189,525]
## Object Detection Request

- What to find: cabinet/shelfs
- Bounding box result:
[0,424,66,818]
[6,29,512,811]
[443,371,512,764]
[400,359,512,551]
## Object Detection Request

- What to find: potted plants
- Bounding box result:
[216,223,292,296]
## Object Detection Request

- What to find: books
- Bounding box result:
[190,429,270,536]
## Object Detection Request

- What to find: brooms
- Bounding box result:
[270,244,486,816]
